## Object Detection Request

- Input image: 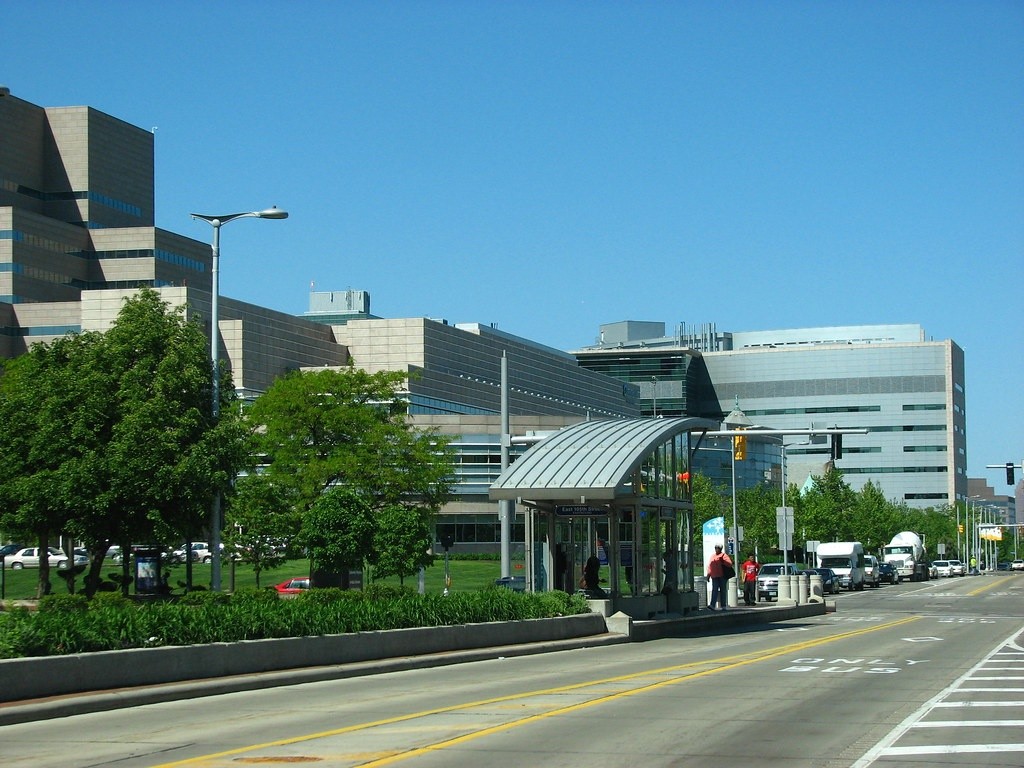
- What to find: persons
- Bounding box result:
[143,566,154,577]
[661,552,674,588]
[741,554,760,606]
[555,544,565,591]
[579,556,608,599]
[705,544,732,611]
[969,555,976,570]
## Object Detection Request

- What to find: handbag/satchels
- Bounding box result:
[720,553,736,579]
[741,569,747,583]
[579,576,586,589]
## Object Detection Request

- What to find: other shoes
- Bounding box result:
[750,602,757,606]
[707,605,715,611]
[722,605,726,612]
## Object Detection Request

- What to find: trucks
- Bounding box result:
[816,541,865,591]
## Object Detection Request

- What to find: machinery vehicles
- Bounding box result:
[881,531,932,582]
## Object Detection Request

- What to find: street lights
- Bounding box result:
[781,442,809,572]
[966,495,1000,575]
[188,203,290,593]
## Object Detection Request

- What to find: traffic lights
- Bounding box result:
[959,525,963,533]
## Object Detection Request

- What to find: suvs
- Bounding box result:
[758,563,799,603]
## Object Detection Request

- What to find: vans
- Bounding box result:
[863,555,879,588]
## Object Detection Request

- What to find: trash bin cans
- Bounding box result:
[135,550,162,593]
[496,576,525,591]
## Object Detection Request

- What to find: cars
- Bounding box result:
[998,562,1013,571]
[927,563,938,579]
[274,577,310,594]
[495,576,526,592]
[948,560,966,576]
[932,560,954,578]
[105,535,309,564]
[4,546,90,570]
[880,562,899,585]
[1011,559,1024,571]
[795,568,840,596]
[0,543,26,562]
